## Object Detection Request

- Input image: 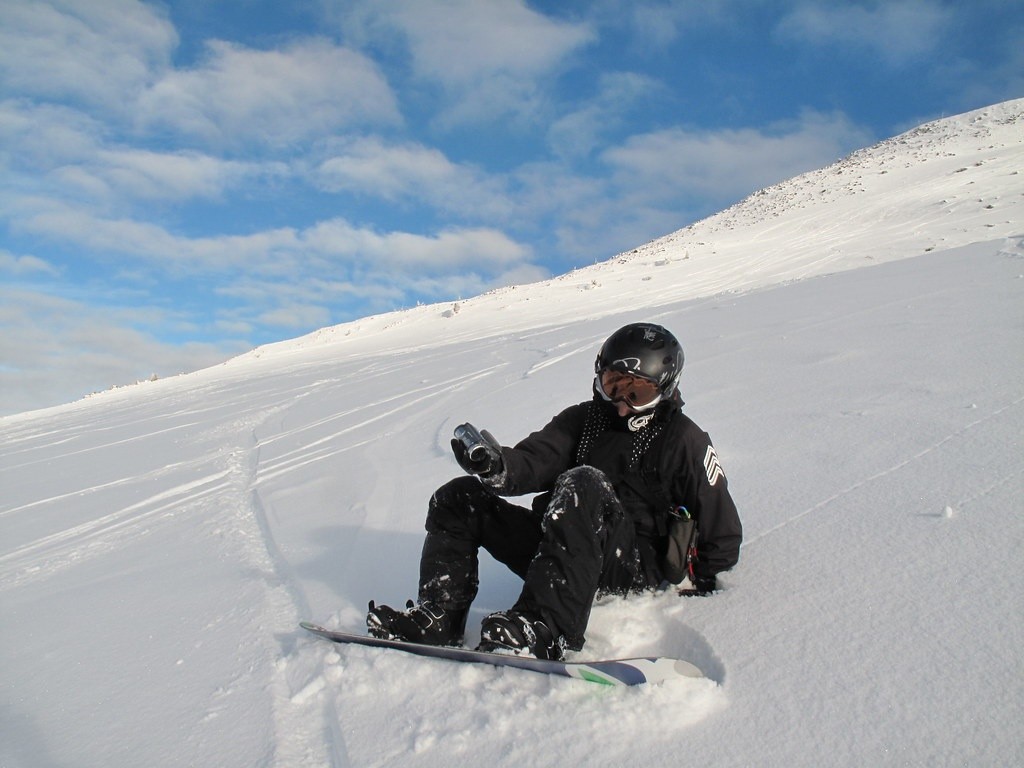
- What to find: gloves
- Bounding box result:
[679,576,717,597]
[450,429,505,479]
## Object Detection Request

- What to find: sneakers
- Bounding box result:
[472,608,567,664]
[365,594,470,650]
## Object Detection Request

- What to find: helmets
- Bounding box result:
[595,322,685,394]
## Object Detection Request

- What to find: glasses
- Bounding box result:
[598,366,666,412]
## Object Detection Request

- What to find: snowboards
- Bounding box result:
[298,619,650,691]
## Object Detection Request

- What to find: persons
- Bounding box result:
[372,324,745,657]
